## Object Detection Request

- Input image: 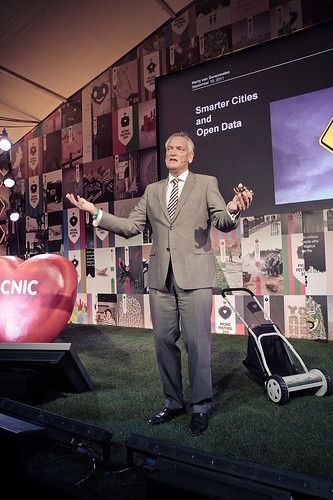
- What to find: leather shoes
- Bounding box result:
[189,406,214,436]
[148,405,187,426]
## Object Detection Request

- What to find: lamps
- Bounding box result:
[2,162,16,188]
[0,128,11,152]
[5,203,19,222]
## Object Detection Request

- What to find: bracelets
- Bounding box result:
[92,208,99,220]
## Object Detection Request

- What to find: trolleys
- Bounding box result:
[220,287,331,406]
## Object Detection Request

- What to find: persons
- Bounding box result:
[65,132,252,437]
[102,309,116,326]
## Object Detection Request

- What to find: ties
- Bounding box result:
[167,178,179,222]
